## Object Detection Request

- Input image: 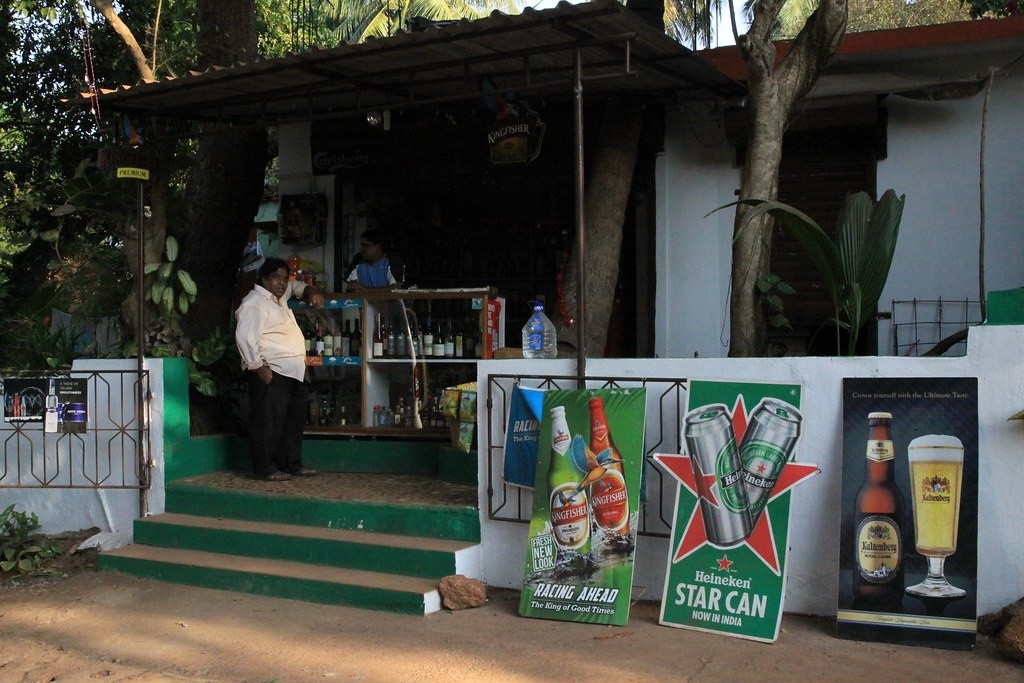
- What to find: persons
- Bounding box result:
[234,256,321,480]
[342,228,407,293]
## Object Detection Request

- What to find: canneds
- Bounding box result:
[682,397,802,550]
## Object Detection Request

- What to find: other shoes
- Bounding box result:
[294,467,320,476]
[253,471,292,481]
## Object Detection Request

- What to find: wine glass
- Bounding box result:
[905,445,967,598]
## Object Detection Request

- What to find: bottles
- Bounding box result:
[521,302,558,358]
[372,314,467,359]
[295,315,362,357]
[303,392,349,427]
[851,411,905,600]
[372,388,450,429]
[44,379,59,432]
[547,405,593,576]
[587,397,631,556]
[4,393,27,417]
[287,250,317,287]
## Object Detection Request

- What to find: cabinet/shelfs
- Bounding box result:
[289,285,505,441]
